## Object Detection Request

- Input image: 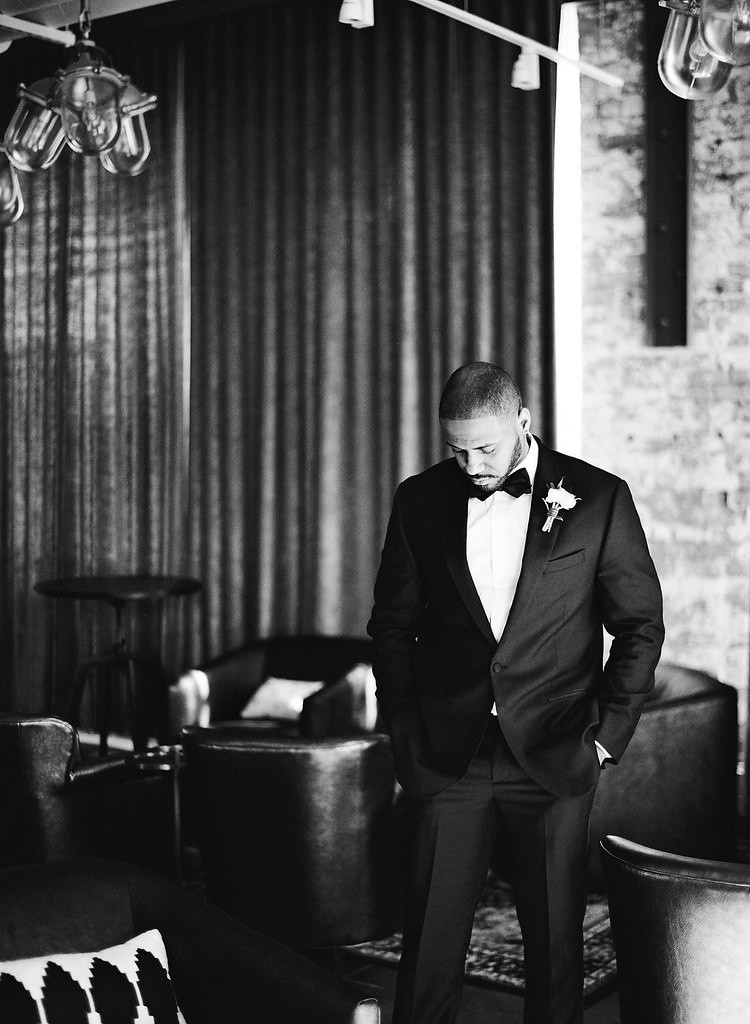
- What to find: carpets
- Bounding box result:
[340,863,622,1009]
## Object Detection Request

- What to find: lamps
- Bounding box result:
[656,0,750,104]
[0,0,158,225]
[340,0,374,29]
[510,48,538,91]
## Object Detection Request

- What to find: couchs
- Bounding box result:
[0,630,750,1024]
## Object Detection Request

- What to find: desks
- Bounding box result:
[33,573,198,782]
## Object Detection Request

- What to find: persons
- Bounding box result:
[366,362,665,1024]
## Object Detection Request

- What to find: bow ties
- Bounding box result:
[466,467,531,502]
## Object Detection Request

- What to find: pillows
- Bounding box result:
[0,927,187,1023]
[238,673,325,724]
